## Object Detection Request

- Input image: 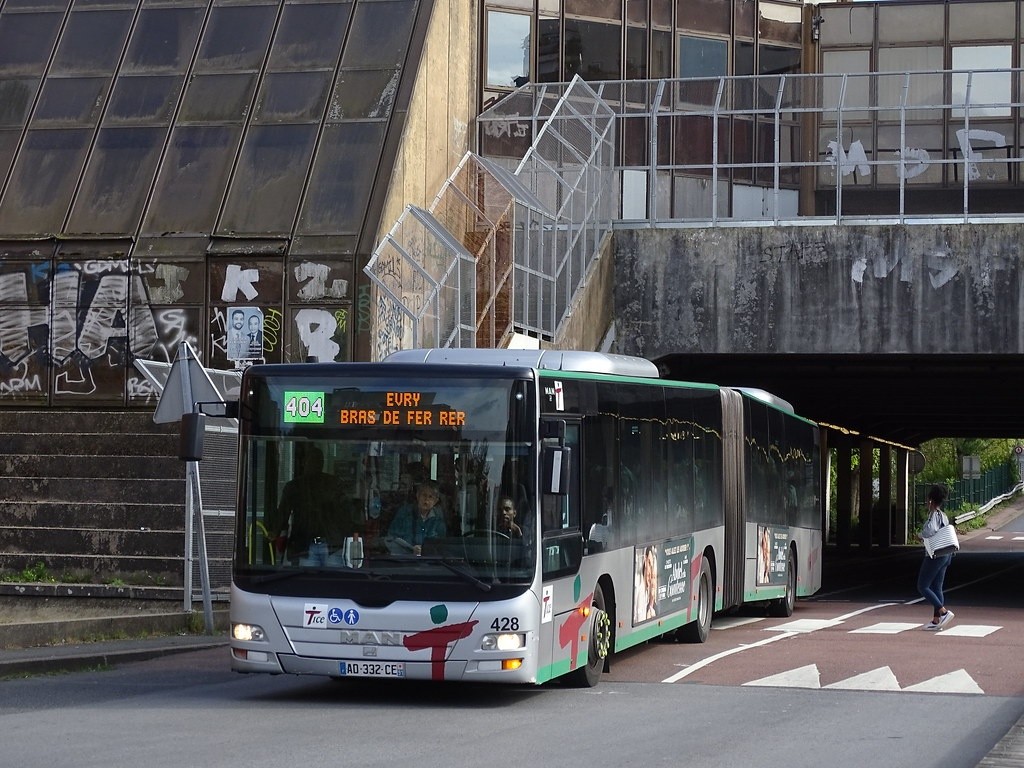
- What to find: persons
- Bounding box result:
[386,482,445,556]
[915,488,956,632]
[634,546,658,622]
[758,527,770,583]
[272,447,352,565]
[494,495,528,540]
[403,462,429,497]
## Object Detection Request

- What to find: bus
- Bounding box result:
[179,347,830,690]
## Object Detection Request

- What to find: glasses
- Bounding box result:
[421,493,436,501]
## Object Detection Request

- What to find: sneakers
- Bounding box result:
[936,610,954,630]
[921,620,942,631]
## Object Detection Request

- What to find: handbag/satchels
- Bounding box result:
[923,508,960,559]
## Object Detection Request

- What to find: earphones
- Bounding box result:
[929,501,931,504]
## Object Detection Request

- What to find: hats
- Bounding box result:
[418,479,441,493]
